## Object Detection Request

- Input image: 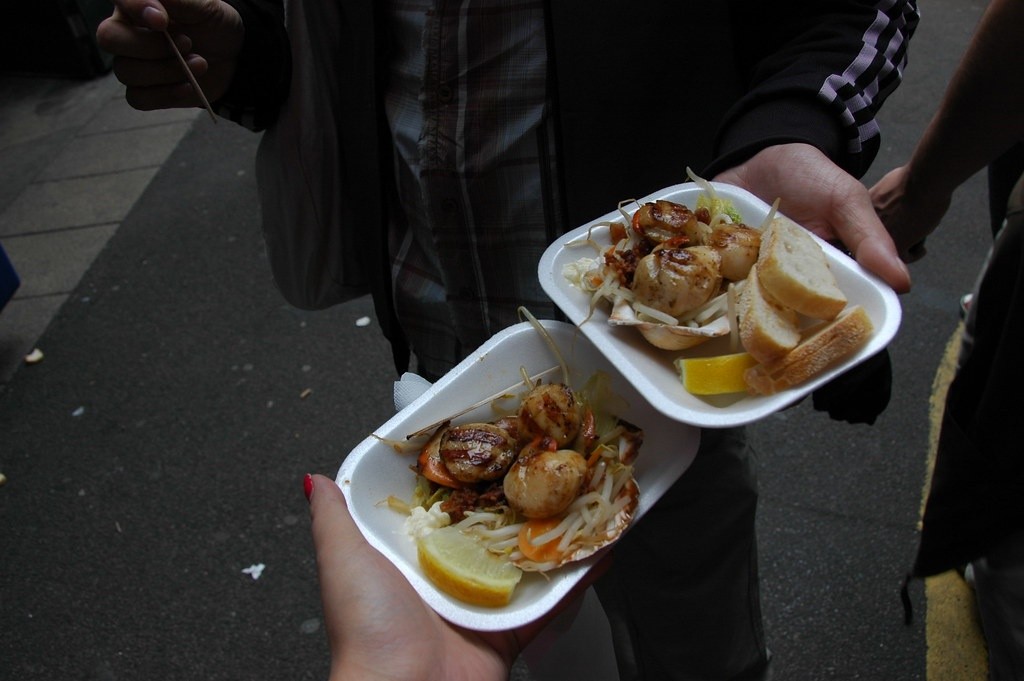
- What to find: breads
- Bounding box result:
[743,303,871,393]
[758,216,847,322]
[740,264,800,364]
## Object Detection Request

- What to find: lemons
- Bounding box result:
[418,531,521,609]
[680,353,752,395]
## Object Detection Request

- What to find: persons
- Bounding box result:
[867,0,1024,680]
[301,472,613,681]
[96,0,919,681]
